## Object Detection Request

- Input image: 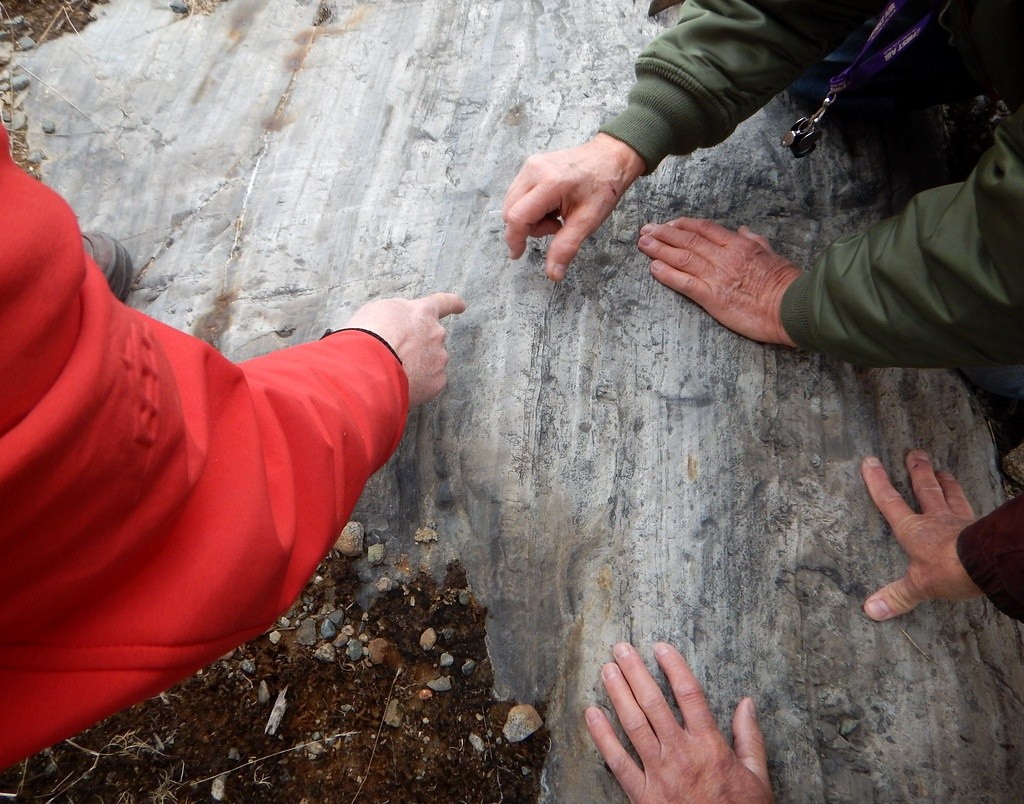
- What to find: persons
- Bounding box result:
[502,0,1024,394]
[0,118,465,769]
[584,451,1024,804]
